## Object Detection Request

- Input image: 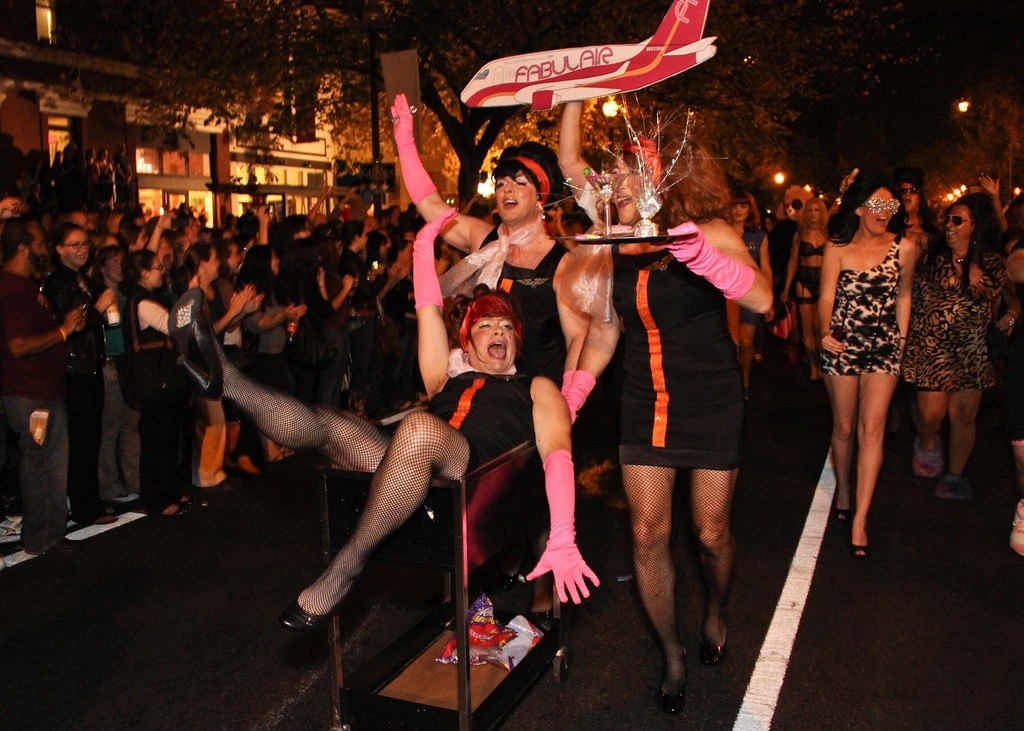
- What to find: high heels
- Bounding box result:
[499,569,520,591]
[528,607,561,623]
[166,287,224,402]
[657,651,689,715]
[698,614,729,666]
[834,506,850,521]
[278,576,358,633]
[849,521,871,560]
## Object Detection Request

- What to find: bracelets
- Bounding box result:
[60,327,67,342]
[900,337,906,339]
[1007,310,1017,326]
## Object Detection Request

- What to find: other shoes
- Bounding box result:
[912,434,944,476]
[934,475,972,500]
[0,453,306,558]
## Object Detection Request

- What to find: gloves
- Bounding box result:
[391,94,437,206]
[560,369,596,424]
[665,222,755,301]
[412,207,461,311]
[525,448,600,604]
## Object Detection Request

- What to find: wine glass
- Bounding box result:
[586,172,629,239]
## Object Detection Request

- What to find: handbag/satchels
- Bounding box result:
[114,291,193,414]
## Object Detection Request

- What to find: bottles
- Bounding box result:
[286,317,297,346]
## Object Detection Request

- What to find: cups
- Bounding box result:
[106,303,120,326]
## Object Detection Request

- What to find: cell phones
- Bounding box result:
[822,326,848,357]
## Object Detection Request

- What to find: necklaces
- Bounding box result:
[958,258,964,263]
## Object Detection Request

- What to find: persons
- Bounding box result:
[0,134,591,553]
[901,174,1024,555]
[168,206,600,633]
[390,92,591,622]
[732,187,840,400]
[563,135,774,715]
[818,176,915,558]
[893,170,932,246]
[560,99,600,223]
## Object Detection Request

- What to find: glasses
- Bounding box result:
[901,187,920,195]
[148,264,164,273]
[61,240,90,251]
[943,215,974,226]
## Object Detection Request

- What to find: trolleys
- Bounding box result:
[313,437,570,730]
